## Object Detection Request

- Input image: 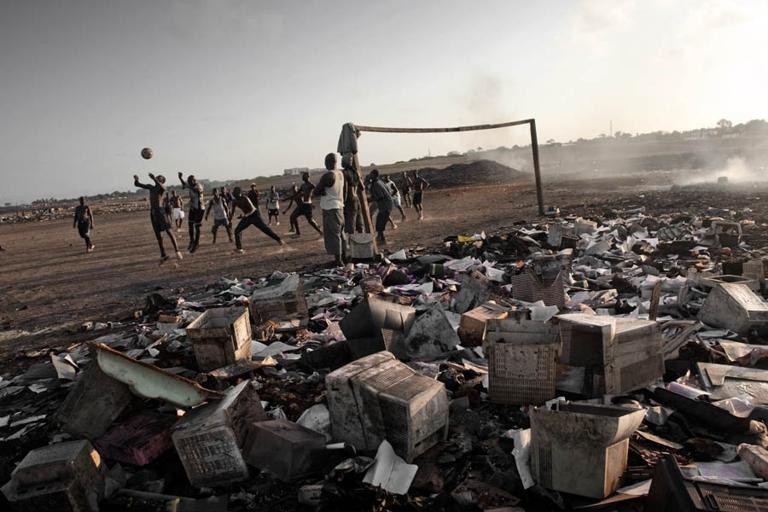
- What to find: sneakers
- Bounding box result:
[329,260,345,267]
[275,222,280,224]
[418,216,424,220]
[268,223,272,225]
[392,224,398,229]
[87,245,95,253]
[188,242,193,249]
[233,249,243,253]
[279,240,284,246]
[159,256,168,262]
[175,251,183,259]
[190,242,200,253]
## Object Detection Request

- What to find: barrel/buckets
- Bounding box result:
[349,232,382,258]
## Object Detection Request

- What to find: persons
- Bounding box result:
[162,169,406,253]
[72,196,95,254]
[399,171,413,208]
[313,153,347,267]
[411,170,430,219]
[134,173,183,262]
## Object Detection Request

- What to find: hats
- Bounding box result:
[250,183,256,186]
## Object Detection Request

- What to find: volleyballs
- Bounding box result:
[141,147,153,159]
